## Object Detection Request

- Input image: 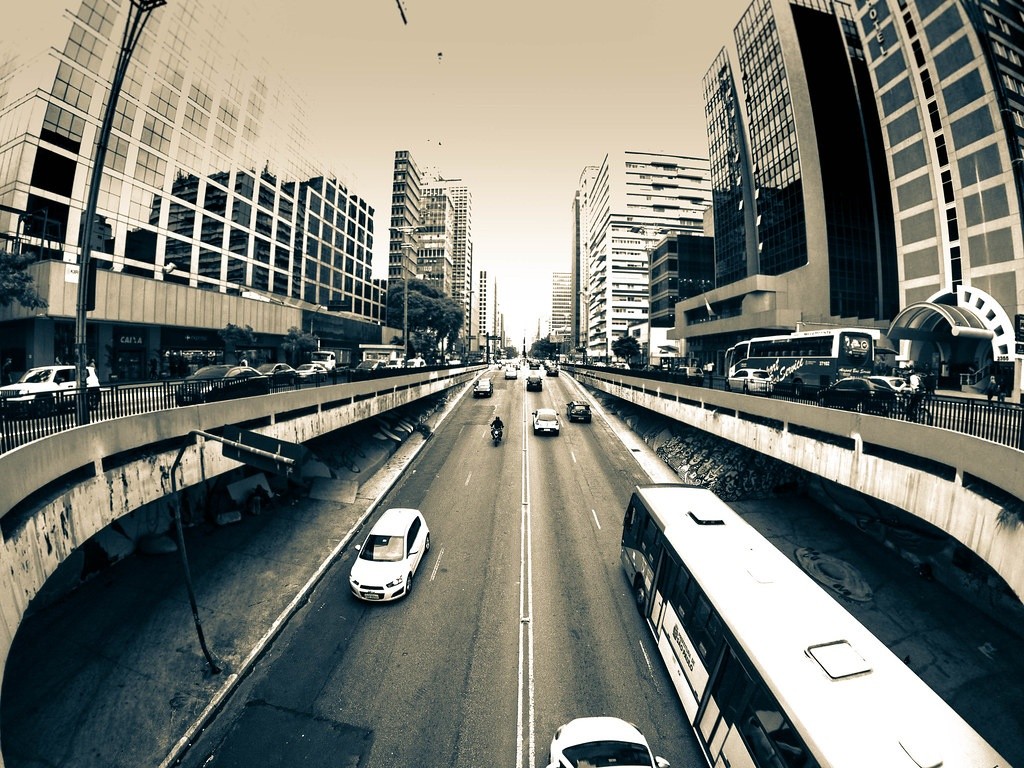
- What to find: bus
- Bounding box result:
[618,475,1015,768]
[726,327,881,399]
[311,351,336,373]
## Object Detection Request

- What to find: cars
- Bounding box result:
[348,507,431,604]
[726,368,775,398]
[337,357,462,370]
[815,376,914,416]
[568,358,705,381]
[473,379,493,398]
[497,357,560,379]
[175,363,327,405]
[545,714,672,768]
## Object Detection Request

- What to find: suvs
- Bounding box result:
[526,375,543,391]
[532,409,560,436]
[0,365,102,419]
[566,400,591,423]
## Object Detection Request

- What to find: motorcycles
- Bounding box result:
[490,424,503,447]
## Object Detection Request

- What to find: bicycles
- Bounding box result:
[887,390,932,425]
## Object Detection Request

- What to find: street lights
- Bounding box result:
[400,225,429,367]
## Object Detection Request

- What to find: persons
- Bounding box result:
[985,376,1009,410]
[490,416,504,441]
[239,355,248,367]
[90,359,98,375]
[706,361,714,381]
[3,358,13,385]
[908,370,937,404]
